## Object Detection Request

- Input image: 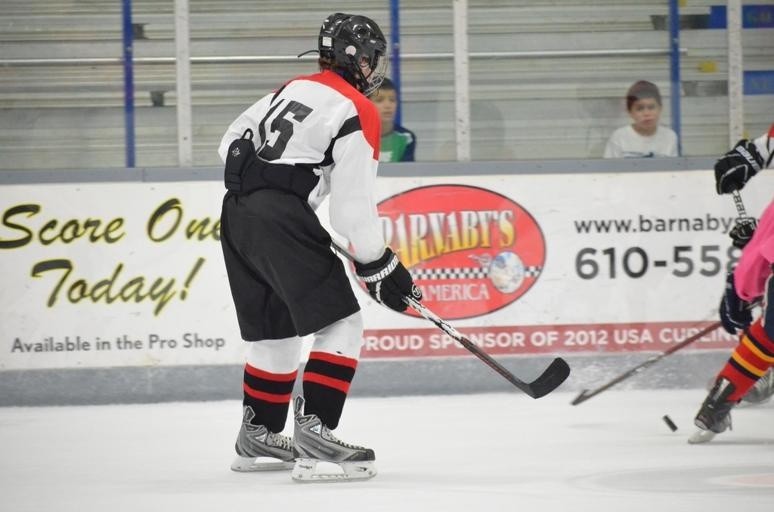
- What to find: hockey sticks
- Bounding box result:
[573,321,723,406]
[331,239,570,399]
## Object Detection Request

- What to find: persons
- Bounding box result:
[602,80,679,160]
[693,202,774,433]
[715,124,773,404]
[218,13,423,462]
[367,75,416,164]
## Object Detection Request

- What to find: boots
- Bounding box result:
[292,395,375,460]
[694,375,736,433]
[235,406,296,461]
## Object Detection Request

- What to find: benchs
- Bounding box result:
[0,1,774,168]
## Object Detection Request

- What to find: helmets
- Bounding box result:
[318,13,390,96]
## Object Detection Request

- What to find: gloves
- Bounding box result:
[720,274,753,334]
[713,137,766,194]
[352,248,422,313]
[729,216,761,248]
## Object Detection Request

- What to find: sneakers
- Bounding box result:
[742,367,774,402]
